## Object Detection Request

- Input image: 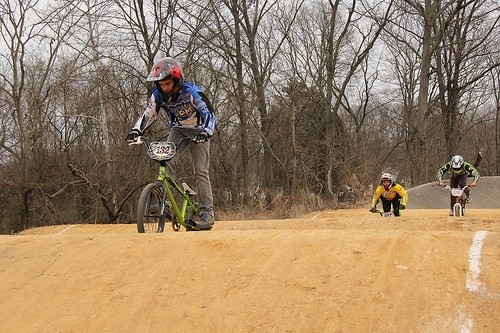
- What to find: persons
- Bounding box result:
[436,155,480,216]
[126,58,215,229]
[371,173,408,216]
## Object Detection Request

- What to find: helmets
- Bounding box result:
[451,155,464,174]
[380,173,393,190]
[146,57,185,102]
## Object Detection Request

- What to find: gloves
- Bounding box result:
[371,208,376,213]
[196,130,210,142]
[401,206,405,209]
[126,129,141,143]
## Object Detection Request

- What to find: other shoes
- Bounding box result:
[449,211,454,216]
[196,213,215,230]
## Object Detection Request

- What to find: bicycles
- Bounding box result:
[439,185,477,217]
[369,209,395,217]
[127,136,200,234]
